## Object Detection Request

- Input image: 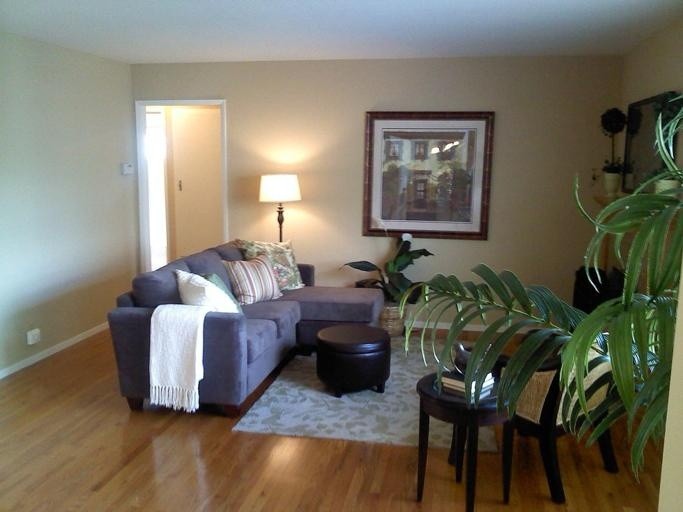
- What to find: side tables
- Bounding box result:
[416,370,515,512]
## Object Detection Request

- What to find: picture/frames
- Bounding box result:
[362,111,495,241]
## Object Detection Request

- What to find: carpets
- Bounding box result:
[232,339,497,451]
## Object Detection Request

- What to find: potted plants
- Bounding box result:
[650,91,683,208]
[337,230,434,340]
[627,107,641,190]
[601,108,626,196]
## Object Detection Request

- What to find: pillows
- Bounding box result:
[175,241,306,315]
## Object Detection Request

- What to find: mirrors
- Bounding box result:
[622,91,676,195]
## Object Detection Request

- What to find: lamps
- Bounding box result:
[259,174,301,242]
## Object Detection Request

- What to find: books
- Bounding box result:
[439,372,496,401]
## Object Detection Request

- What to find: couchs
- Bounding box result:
[106,239,383,417]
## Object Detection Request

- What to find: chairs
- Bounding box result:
[449,266,625,503]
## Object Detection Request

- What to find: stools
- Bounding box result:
[318,322,392,397]
